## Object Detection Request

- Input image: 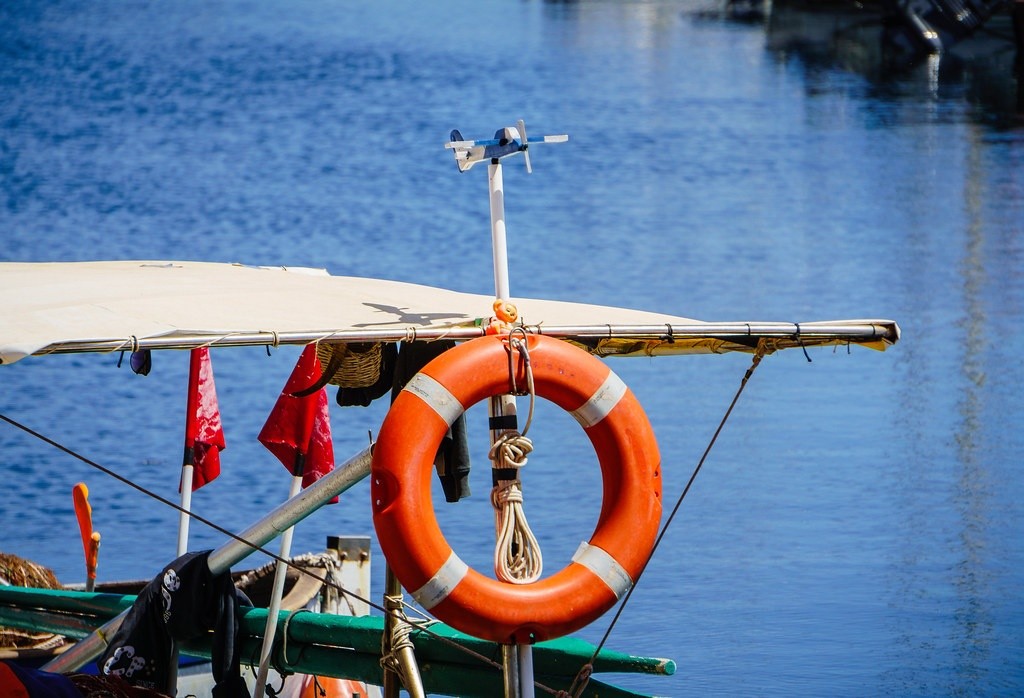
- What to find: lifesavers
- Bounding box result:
[369,332,663,645]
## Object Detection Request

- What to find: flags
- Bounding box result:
[178,346,227,494]
[257,345,340,505]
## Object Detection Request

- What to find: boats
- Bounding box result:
[1,115,900,698]
